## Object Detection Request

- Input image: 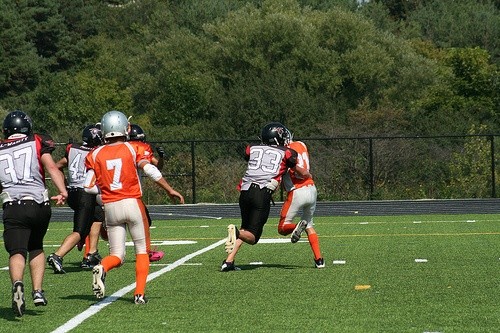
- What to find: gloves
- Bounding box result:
[155,147,164,158]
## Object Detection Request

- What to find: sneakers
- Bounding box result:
[147,251,163,260]
[31,291,47,306]
[134,295,146,305]
[80,261,92,268]
[224,224,239,255]
[46,252,65,273]
[11,281,25,317]
[314,258,325,268]
[92,264,106,299]
[290,220,308,244]
[87,253,103,264]
[220,260,241,273]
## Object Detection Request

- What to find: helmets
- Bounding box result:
[101,111,127,136]
[130,124,144,140]
[283,129,293,144]
[262,122,287,146]
[82,124,104,146]
[3,111,32,137]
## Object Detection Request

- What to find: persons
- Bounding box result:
[222,122,325,272]
[46,123,164,274]
[83,111,185,304]
[0,110,68,316]
